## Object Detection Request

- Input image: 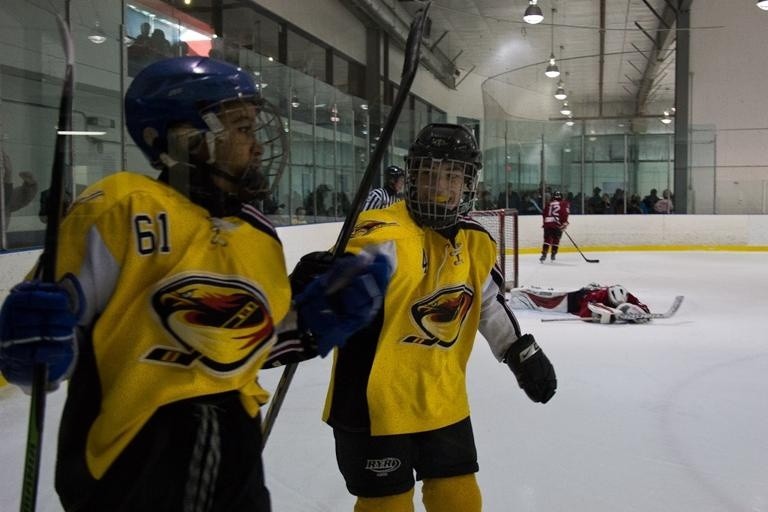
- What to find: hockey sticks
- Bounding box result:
[258,0,429,450]
[552,212,599,262]
[19,0,75,512]
[540,296,684,323]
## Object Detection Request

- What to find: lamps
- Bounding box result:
[555,81,575,127]
[290,85,300,108]
[88,8,106,45]
[252,54,269,89]
[524,0,544,23]
[330,101,339,122]
[756,0,768,12]
[545,8,558,78]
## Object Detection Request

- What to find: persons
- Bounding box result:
[248,165,406,216]
[540,191,568,261]
[128,23,239,79]
[289,124,556,512]
[474,182,674,215]
[0,150,74,251]
[0,56,394,512]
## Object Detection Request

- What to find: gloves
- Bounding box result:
[289,251,391,356]
[503,333,557,404]
[0,272,86,385]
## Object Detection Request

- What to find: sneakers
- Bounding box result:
[540,256,555,260]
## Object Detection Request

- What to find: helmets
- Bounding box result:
[404,124,482,230]
[126,56,288,202]
[386,166,403,190]
[552,190,562,199]
